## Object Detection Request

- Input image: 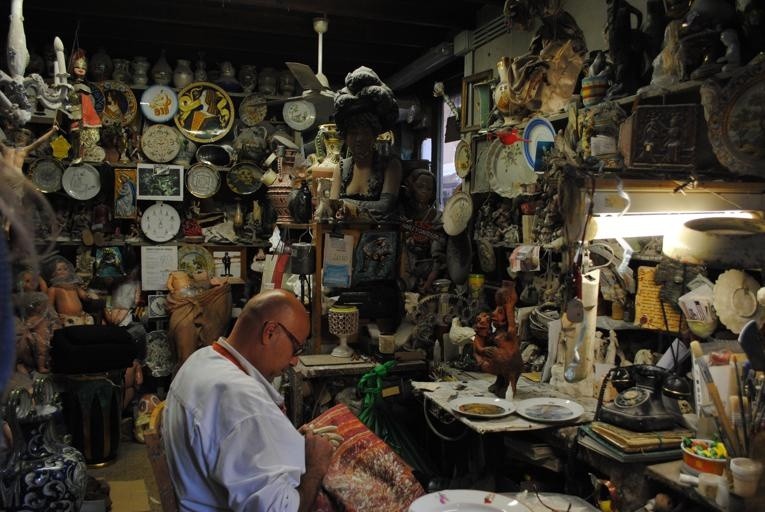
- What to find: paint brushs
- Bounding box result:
[689,340,765,459]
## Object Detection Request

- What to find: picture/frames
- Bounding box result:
[210,245,247,284]
[106,163,140,220]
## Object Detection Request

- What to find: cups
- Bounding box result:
[522,214,534,244]
[679,438,727,477]
[716,412,765,462]
[728,457,764,496]
[581,78,607,108]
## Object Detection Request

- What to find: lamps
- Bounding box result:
[326,307,359,359]
[558,175,763,245]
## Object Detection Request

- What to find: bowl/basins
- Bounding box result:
[528,306,558,341]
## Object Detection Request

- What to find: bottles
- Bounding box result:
[266,149,300,223]
[310,123,345,209]
[70,47,297,97]
[611,300,634,322]
[494,61,522,127]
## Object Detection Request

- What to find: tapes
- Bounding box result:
[264,153,276,167]
[260,169,277,186]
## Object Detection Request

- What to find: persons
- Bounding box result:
[221,251,233,276]
[716,29,743,73]
[602,1,646,101]
[310,65,403,221]
[47,259,101,325]
[164,288,345,512]
[648,1,718,88]
[404,166,448,289]
[15,269,49,375]
[57,49,93,166]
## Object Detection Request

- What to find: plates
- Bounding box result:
[447,396,516,418]
[175,245,214,281]
[707,66,765,178]
[408,489,535,512]
[713,269,765,336]
[227,162,265,195]
[522,117,557,175]
[485,135,539,198]
[185,163,221,199]
[515,397,584,423]
[139,85,180,163]
[28,157,101,201]
[144,331,173,378]
[442,192,474,236]
[454,140,472,178]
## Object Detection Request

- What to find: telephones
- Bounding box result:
[599,364,691,433]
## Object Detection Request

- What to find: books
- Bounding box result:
[577,425,686,463]
[504,433,553,460]
[591,420,693,452]
[539,458,560,472]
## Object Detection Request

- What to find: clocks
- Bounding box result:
[30,157,64,195]
[62,163,101,201]
[140,124,180,164]
[148,294,168,319]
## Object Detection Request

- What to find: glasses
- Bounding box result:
[263,319,303,356]
